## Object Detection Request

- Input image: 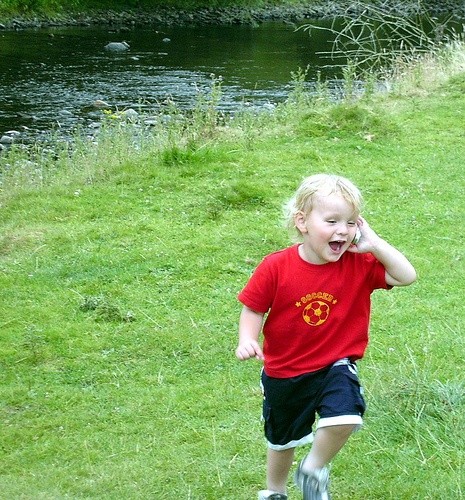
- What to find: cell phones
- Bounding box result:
[352,224,361,245]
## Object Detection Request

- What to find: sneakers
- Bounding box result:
[258,489,287,500]
[294,454,332,499]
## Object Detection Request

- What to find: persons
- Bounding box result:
[234,172,416,499]
